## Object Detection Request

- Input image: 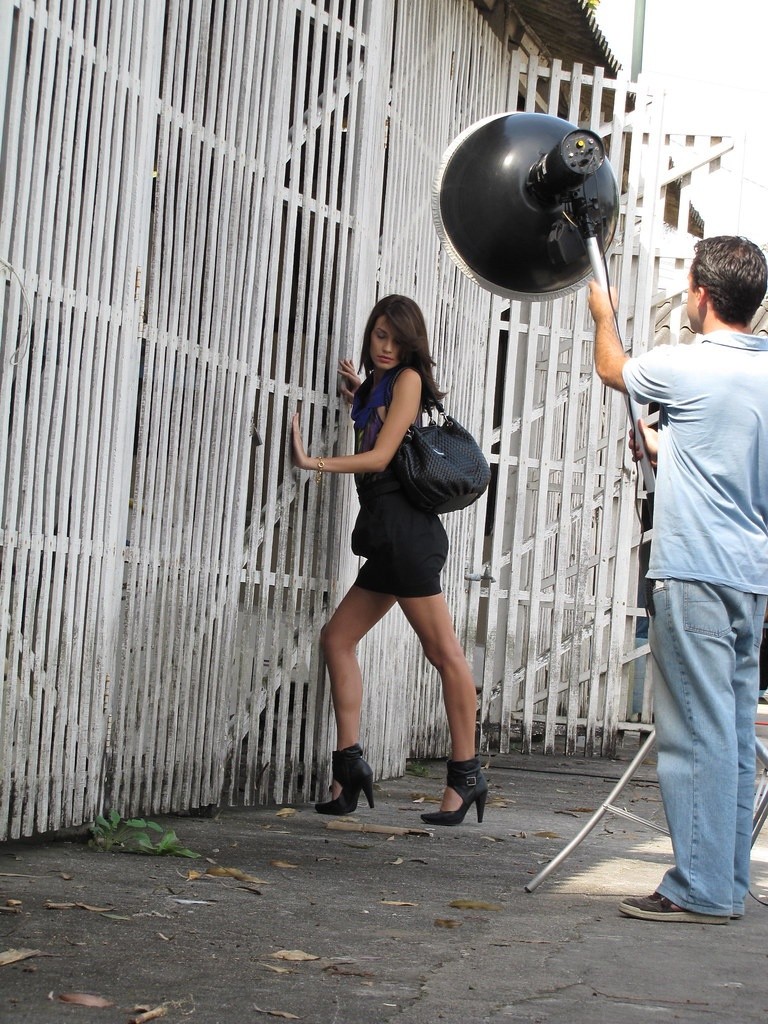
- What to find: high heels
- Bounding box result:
[420,757,488,825]
[314,744,374,816]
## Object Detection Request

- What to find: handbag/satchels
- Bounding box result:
[386,366,492,513]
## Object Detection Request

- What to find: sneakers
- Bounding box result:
[618,890,740,924]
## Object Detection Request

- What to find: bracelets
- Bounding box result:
[315,456,324,484]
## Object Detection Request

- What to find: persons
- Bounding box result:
[291,293,488,826]
[587,235,768,926]
